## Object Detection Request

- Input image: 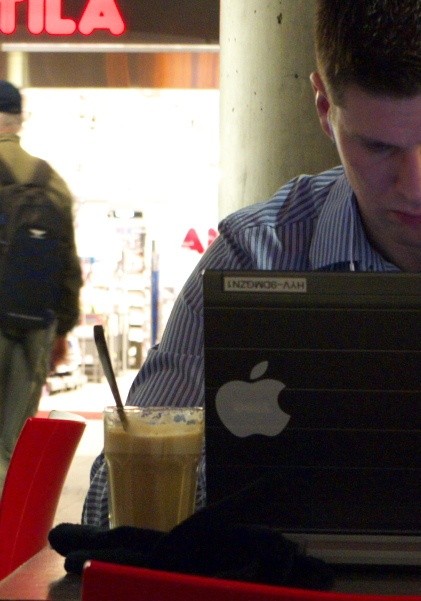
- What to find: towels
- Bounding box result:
[47,466,334,590]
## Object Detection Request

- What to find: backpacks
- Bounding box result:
[0,157,72,337]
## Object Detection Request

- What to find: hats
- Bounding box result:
[0,80,20,113]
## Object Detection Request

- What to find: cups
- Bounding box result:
[103,405,206,533]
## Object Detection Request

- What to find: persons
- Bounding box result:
[80,4,421,525]
[0,70,81,499]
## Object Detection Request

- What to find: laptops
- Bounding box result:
[203,269,420,534]
